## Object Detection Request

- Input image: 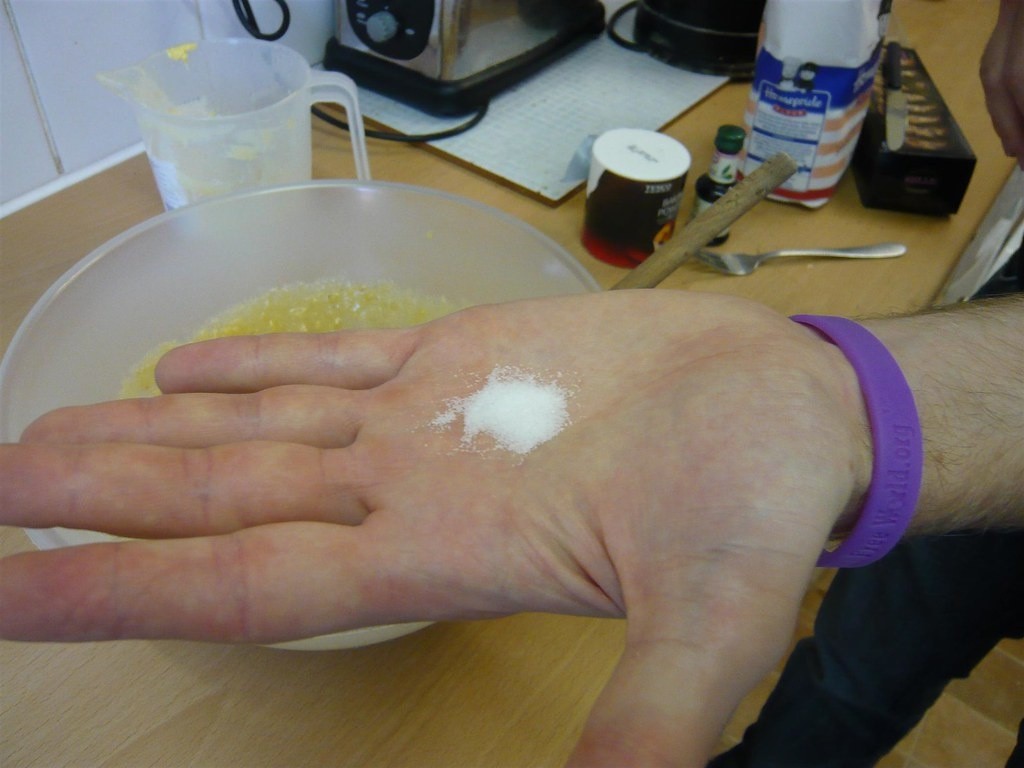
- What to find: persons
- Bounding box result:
[0,0,1024,768]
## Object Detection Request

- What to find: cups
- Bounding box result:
[582,126,691,270]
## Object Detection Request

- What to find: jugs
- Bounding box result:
[94,37,368,211]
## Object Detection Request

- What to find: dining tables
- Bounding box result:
[0,1,1019,768]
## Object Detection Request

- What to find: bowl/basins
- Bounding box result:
[1,178,603,652]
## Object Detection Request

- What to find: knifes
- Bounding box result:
[884,41,907,151]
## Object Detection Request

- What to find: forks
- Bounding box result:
[686,237,906,277]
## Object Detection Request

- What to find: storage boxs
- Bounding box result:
[851,46,978,219]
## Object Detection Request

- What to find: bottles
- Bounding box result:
[694,125,746,246]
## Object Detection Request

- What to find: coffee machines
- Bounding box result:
[324,0,606,118]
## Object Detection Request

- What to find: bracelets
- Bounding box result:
[789,314,922,568]
